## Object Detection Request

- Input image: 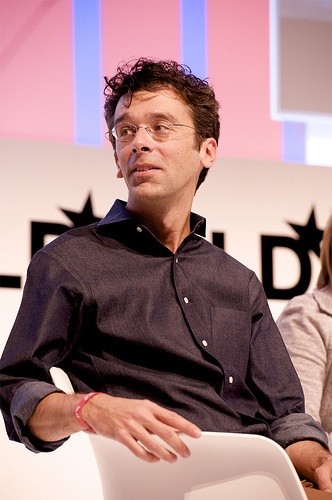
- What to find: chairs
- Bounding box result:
[49,368,310,500]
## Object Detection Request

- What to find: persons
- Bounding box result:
[0,55,332,492]
[275,214,332,451]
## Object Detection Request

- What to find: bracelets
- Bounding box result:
[74,390,108,435]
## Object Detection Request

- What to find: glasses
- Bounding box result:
[111,118,201,142]
